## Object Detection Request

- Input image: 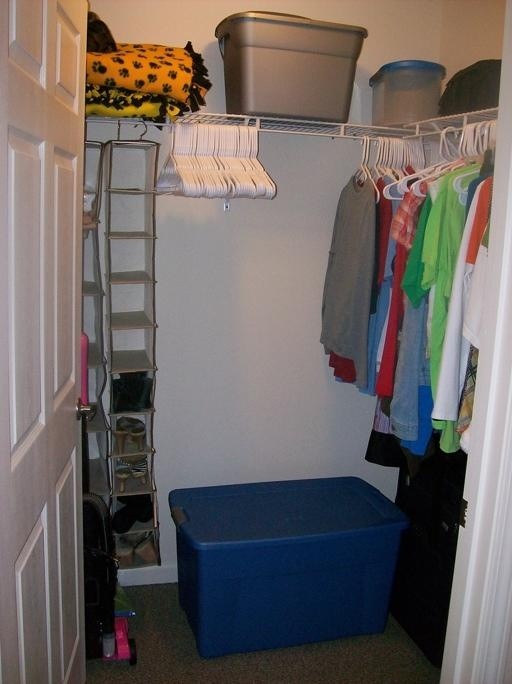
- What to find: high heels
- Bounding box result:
[112,418,146,455]
[115,461,148,492]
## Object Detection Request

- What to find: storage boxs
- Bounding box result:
[214,11,367,123]
[369,60,447,125]
[167,477,409,659]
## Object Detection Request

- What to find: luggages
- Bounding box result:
[82,492,117,660]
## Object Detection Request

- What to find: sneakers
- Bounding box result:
[114,494,156,565]
